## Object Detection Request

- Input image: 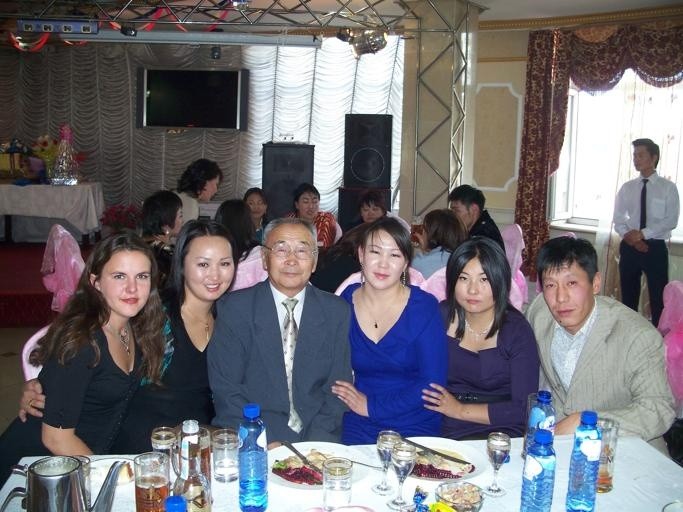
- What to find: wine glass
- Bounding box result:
[482,431,510,498]
[371,428,415,512]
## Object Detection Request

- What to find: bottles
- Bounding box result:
[235,404,269,512]
[564,411,601,512]
[522,390,555,512]
[173,418,212,512]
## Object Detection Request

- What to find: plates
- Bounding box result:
[405,437,482,481]
[270,440,367,492]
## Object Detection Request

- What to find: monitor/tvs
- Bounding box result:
[136,65,249,132]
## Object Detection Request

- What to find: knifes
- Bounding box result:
[403,437,472,465]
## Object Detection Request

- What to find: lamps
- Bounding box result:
[119,22,137,37]
[353,26,389,55]
[60,29,325,47]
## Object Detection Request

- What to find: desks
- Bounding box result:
[0,179,105,250]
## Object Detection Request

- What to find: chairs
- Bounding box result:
[232,244,273,290]
[334,266,425,301]
[500,222,530,305]
[659,277,682,401]
[21,322,55,382]
[42,223,89,313]
[420,267,522,313]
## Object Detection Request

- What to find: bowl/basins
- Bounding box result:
[434,481,484,512]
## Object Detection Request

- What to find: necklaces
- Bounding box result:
[465,319,496,345]
[359,287,407,331]
[184,304,212,342]
[97,316,136,353]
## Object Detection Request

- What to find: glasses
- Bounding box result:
[264,243,317,260]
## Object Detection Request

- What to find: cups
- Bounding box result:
[320,454,352,510]
[178,427,211,477]
[590,417,617,494]
[132,427,175,511]
[211,428,239,481]
[75,455,93,507]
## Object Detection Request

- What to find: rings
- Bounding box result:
[27,398,35,406]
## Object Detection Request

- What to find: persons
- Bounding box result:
[137,188,185,284]
[448,183,504,251]
[17,218,240,452]
[208,217,353,447]
[213,200,270,291]
[358,189,388,225]
[243,186,272,243]
[526,237,679,465]
[332,216,446,443]
[1,227,164,494]
[407,206,464,279]
[309,221,372,294]
[277,184,336,247]
[171,157,223,227]
[422,235,539,437]
[610,138,681,328]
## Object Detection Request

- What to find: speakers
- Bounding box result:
[345,114,392,189]
[261,144,314,223]
[338,188,391,235]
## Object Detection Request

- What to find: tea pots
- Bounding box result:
[0,454,128,512]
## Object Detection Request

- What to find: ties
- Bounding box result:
[282,300,304,434]
[640,179,648,229]
